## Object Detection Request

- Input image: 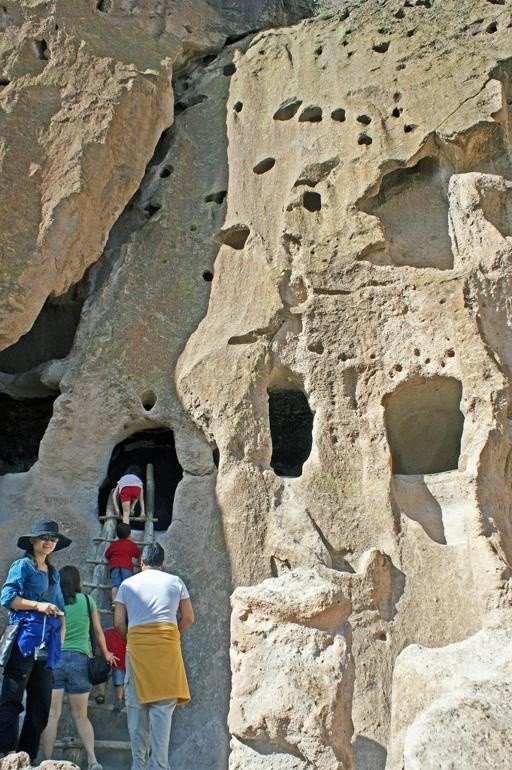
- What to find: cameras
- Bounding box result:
[50,610,65,617]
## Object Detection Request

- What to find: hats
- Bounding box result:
[16,520,72,552]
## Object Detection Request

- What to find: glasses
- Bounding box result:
[40,534,58,542]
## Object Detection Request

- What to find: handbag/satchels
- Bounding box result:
[88,656,109,686]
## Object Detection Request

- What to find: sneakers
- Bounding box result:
[95,694,125,713]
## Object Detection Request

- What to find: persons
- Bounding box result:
[42,566,121,770]
[94,627,127,712]
[112,470,146,525]
[113,541,195,770]
[0,518,72,764]
[105,523,141,611]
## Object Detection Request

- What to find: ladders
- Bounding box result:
[56,463,159,768]
